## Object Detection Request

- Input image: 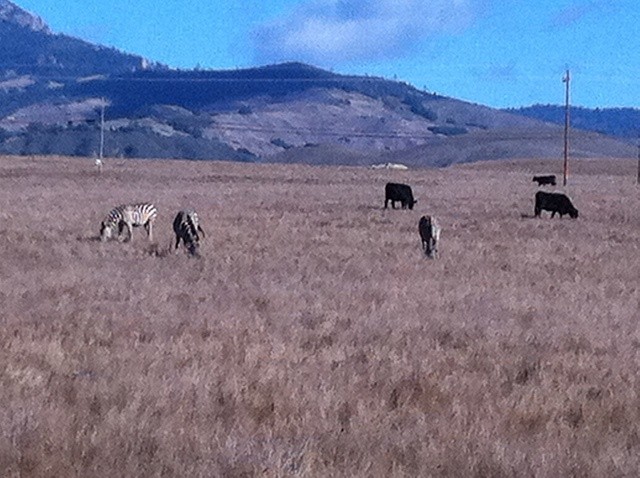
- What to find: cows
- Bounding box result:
[535,191,578,219]
[385,182,417,210]
[532,175,556,186]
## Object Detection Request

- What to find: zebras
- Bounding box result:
[173,209,206,259]
[99,202,159,242]
[419,215,442,257]
